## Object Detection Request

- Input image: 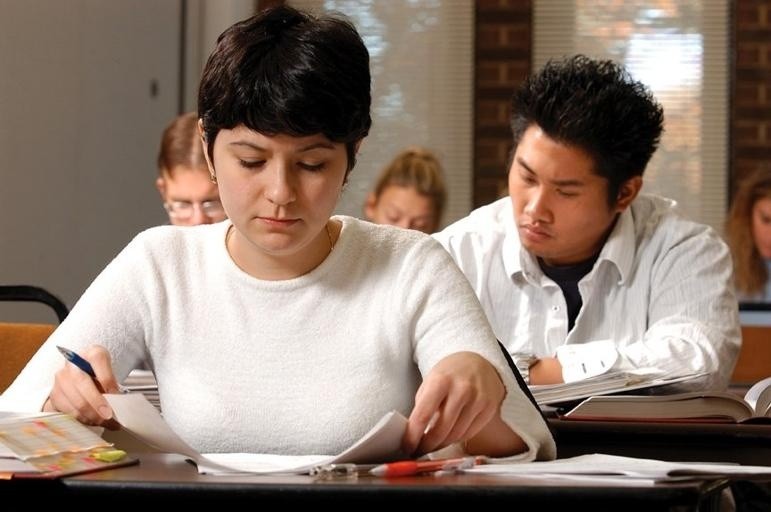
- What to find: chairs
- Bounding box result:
[0,285,68,395]
[729,302,771,387]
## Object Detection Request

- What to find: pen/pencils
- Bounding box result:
[310,464,377,477]
[56,345,130,393]
[369,454,486,478]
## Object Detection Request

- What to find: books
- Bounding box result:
[518,364,715,406]
[1,412,140,477]
[124,370,159,413]
[561,378,770,422]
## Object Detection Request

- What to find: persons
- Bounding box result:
[154,111,227,226]
[716,158,770,327]
[430,49,743,394]
[364,146,448,236]
[0,7,557,474]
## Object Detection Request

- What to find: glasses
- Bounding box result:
[163,199,224,218]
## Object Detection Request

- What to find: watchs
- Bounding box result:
[510,352,538,386]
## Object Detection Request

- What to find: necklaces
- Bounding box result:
[224,222,334,282]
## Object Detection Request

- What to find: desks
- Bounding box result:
[541,392,770,468]
[1,450,742,512]
